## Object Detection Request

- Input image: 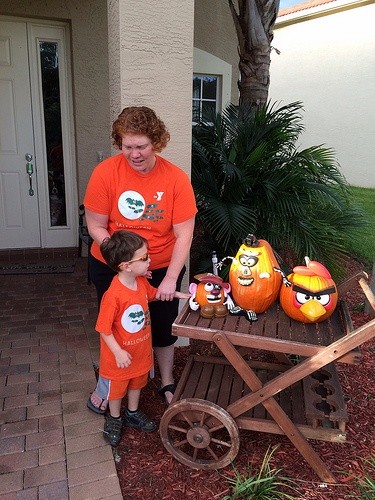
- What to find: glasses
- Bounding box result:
[117,253,148,267]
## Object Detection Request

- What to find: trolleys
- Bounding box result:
[157,269,375,484]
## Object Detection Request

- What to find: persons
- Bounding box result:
[94,230,192,446]
[83,105,198,415]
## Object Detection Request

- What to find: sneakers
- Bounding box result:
[123,409,156,432]
[103,414,123,446]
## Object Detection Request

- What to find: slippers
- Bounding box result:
[87,392,109,414]
[158,383,177,406]
